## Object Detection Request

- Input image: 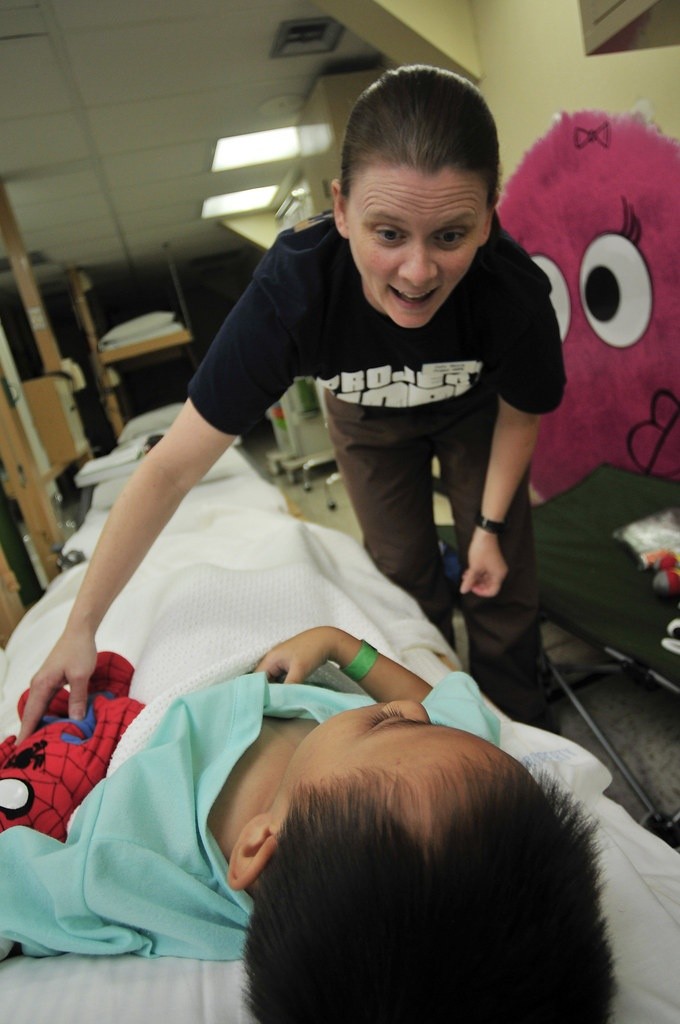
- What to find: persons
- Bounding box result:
[14,63,564,746]
[1,628,617,1024]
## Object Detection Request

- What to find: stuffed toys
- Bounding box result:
[1,652,146,844]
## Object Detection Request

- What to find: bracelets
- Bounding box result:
[338,637,378,683]
[477,513,505,534]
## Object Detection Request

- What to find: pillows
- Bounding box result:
[116,403,185,443]
[99,310,176,344]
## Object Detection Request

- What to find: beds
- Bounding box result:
[98,323,198,423]
[0,399,680,1024]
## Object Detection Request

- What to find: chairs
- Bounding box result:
[434,388,679,852]
[302,379,343,510]
